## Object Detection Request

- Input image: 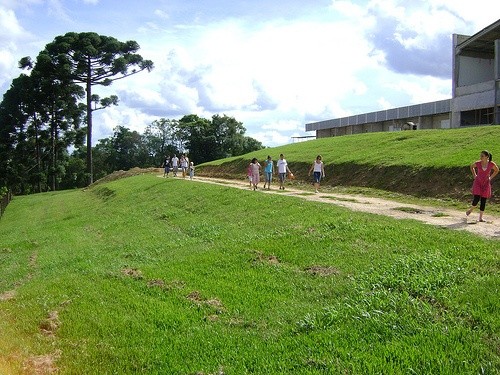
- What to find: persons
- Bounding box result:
[180,153,189,177]
[190,161,195,181]
[247,163,253,187]
[308,156,325,193]
[264,155,274,190]
[277,154,293,190]
[161,162,166,177]
[164,156,172,178]
[464,150,499,222]
[249,158,262,190]
[171,153,180,177]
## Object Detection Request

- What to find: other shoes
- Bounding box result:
[256,186,258,189]
[466,209,472,216]
[264,184,266,188]
[267,187,271,190]
[478,217,485,222]
[279,186,282,190]
[253,188,255,191]
[282,187,285,190]
[314,189,319,193]
